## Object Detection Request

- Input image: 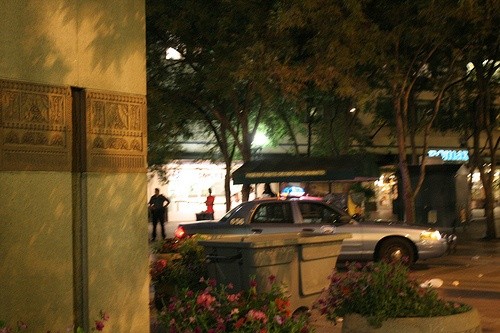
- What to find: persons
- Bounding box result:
[227,182,281,210]
[148,187,171,241]
[204,188,215,215]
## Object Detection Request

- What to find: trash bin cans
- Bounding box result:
[197,232,353,322]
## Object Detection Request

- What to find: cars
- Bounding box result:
[175,199,455,268]
